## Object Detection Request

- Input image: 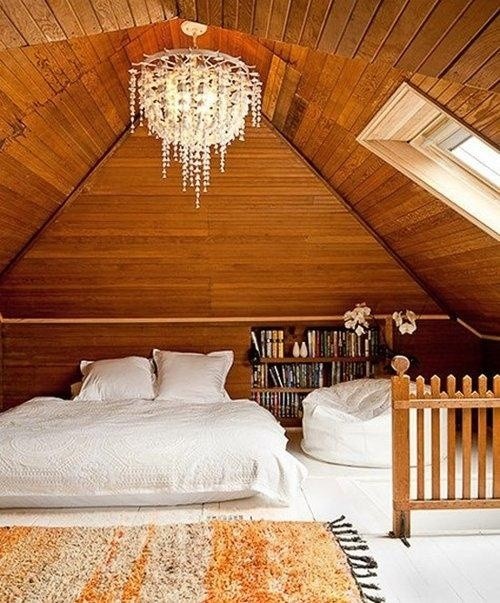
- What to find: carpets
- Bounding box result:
[0,515,386,603]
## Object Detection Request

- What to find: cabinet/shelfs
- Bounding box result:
[249,320,393,424]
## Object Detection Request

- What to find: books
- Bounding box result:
[250,327,380,419]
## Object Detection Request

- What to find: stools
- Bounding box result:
[300,376,454,467]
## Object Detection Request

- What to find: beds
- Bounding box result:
[1,396,308,507]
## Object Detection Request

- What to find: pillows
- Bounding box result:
[78,355,158,407]
[152,349,234,407]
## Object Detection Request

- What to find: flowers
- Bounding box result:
[342,303,421,338]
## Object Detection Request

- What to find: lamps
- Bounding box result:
[125,21,266,209]
